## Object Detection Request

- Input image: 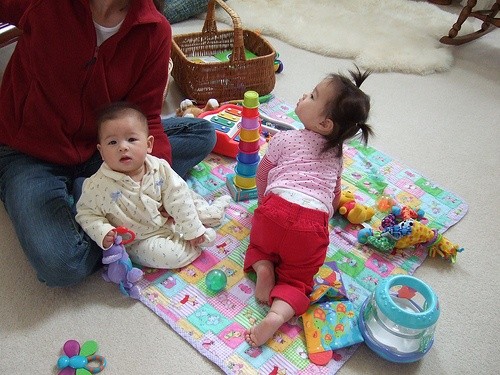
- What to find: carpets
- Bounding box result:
[200,2,473,76]
[139,96,470,375]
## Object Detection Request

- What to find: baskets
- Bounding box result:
[171,0,276,109]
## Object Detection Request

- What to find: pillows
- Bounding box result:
[160,0,218,26]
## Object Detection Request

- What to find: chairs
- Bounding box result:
[438,0,500,46]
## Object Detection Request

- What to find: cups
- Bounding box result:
[358,275,438,363]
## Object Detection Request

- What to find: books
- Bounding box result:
[301,261,364,354]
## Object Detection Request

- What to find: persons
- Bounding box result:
[0,0,215,288]
[75,107,225,269]
[243,62,374,345]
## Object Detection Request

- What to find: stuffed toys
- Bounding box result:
[176,99,219,119]
[101,236,143,300]
[339,190,374,224]
[357,198,465,265]
